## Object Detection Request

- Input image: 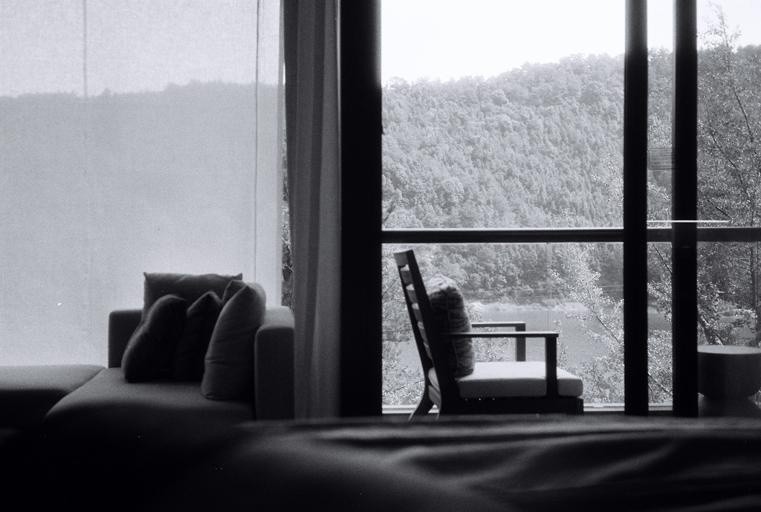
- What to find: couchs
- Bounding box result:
[36,272,297,487]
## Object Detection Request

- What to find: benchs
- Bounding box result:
[0,362,106,432]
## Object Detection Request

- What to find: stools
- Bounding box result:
[696,344,761,416]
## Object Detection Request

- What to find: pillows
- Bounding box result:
[185,286,221,357]
[120,293,188,385]
[141,273,241,317]
[200,279,267,402]
[425,273,475,376]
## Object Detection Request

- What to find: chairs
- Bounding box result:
[393,247,586,423]
